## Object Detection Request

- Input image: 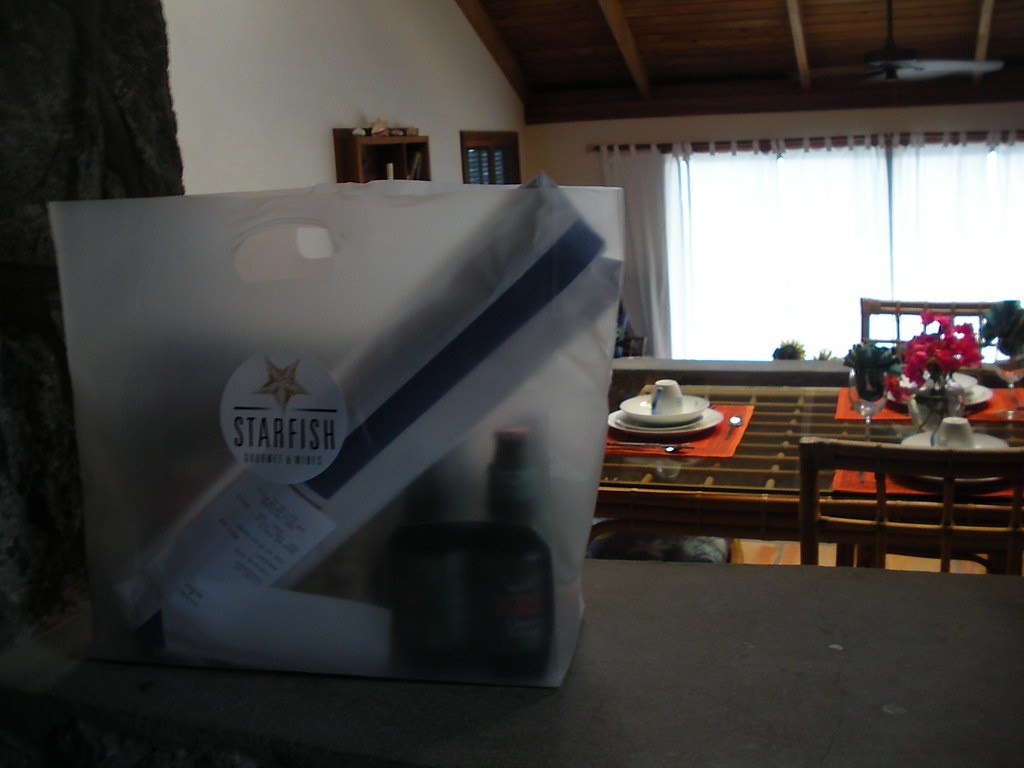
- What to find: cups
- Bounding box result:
[938,418,976,450]
[653,379,683,414]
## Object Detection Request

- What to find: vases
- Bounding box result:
[908,374,966,432]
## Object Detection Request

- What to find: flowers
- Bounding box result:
[902,310,984,388]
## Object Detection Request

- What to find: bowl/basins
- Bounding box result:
[900,375,978,404]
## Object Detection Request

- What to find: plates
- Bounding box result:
[607,410,724,435]
[900,431,1008,448]
[620,395,710,423]
[888,385,993,408]
[615,414,704,429]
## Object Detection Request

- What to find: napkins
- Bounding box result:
[980,301,1024,356]
[844,343,902,402]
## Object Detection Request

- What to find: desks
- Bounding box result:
[585,384,1024,577]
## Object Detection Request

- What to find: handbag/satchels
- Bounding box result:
[47,180,625,688]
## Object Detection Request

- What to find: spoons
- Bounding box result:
[725,415,744,441]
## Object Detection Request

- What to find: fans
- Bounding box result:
[792,0,1003,82]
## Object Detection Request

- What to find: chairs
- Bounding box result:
[799,436,1024,576]
[861,298,1024,363]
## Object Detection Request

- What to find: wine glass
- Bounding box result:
[846,368,887,441]
[996,348,1024,419]
